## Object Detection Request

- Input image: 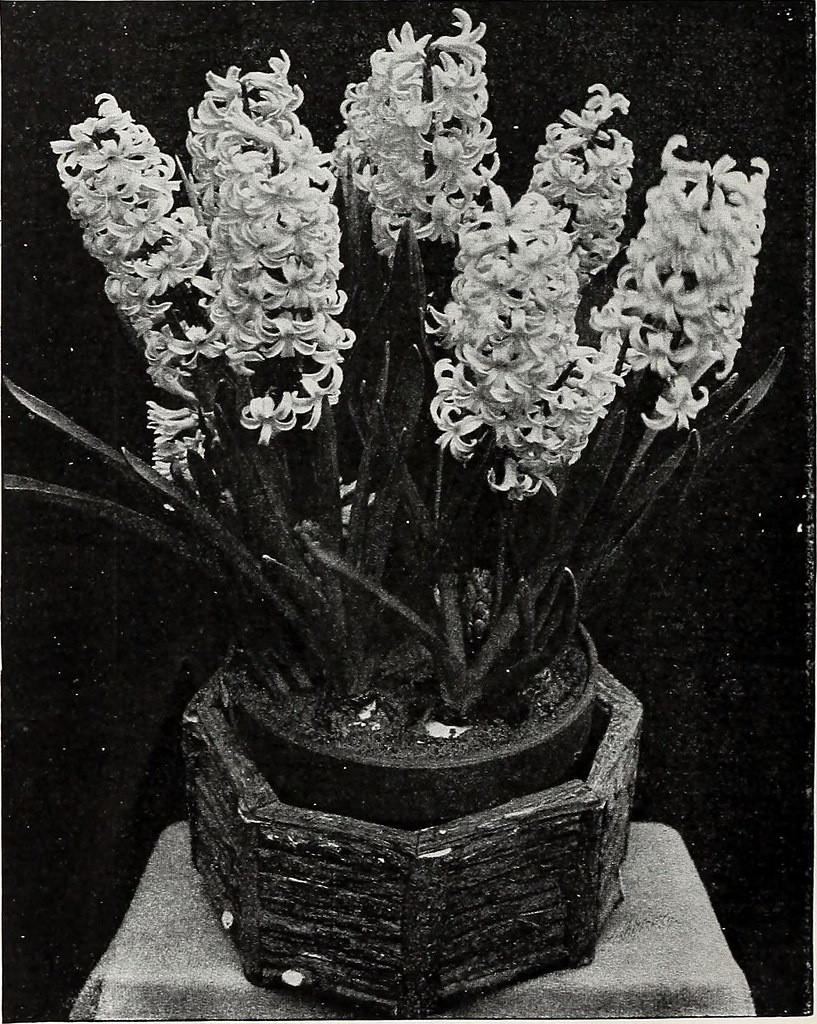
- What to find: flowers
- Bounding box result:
[1,9,787,720]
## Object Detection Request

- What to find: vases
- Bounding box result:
[216,627,601,827]
